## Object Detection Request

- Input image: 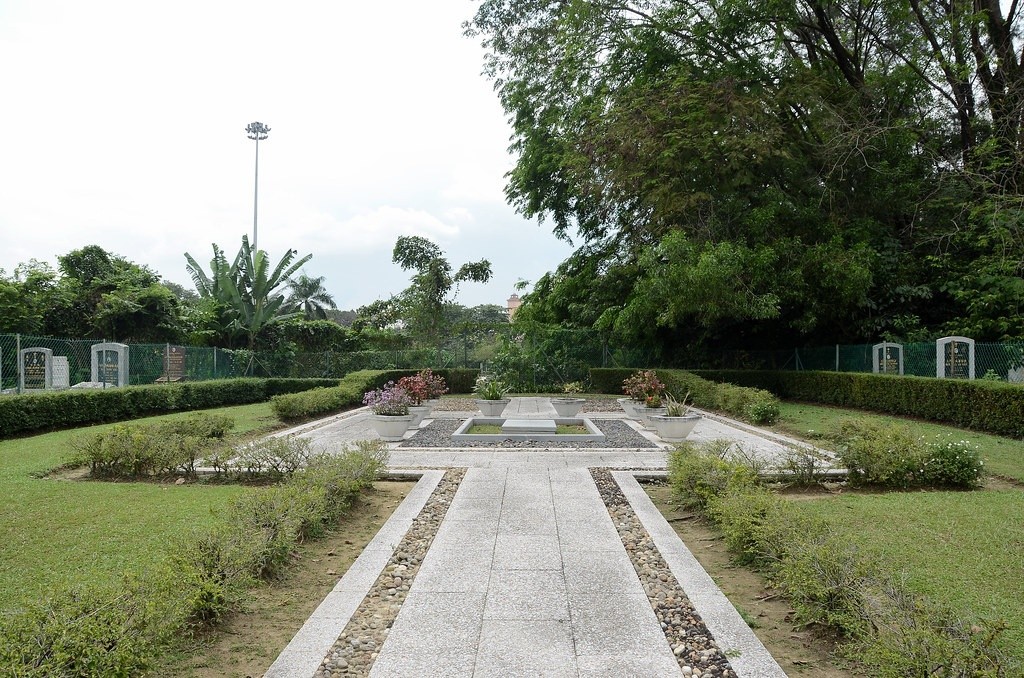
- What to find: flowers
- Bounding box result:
[420,367,449,399]
[397,372,428,407]
[361,381,412,415]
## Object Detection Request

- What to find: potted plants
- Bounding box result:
[471,371,512,417]
[616,370,666,420]
[633,394,675,430]
[549,381,586,417]
[648,390,702,441]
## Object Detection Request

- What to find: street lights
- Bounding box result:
[243,118,274,281]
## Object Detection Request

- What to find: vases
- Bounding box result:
[373,413,419,442]
[421,399,439,418]
[404,406,431,430]
